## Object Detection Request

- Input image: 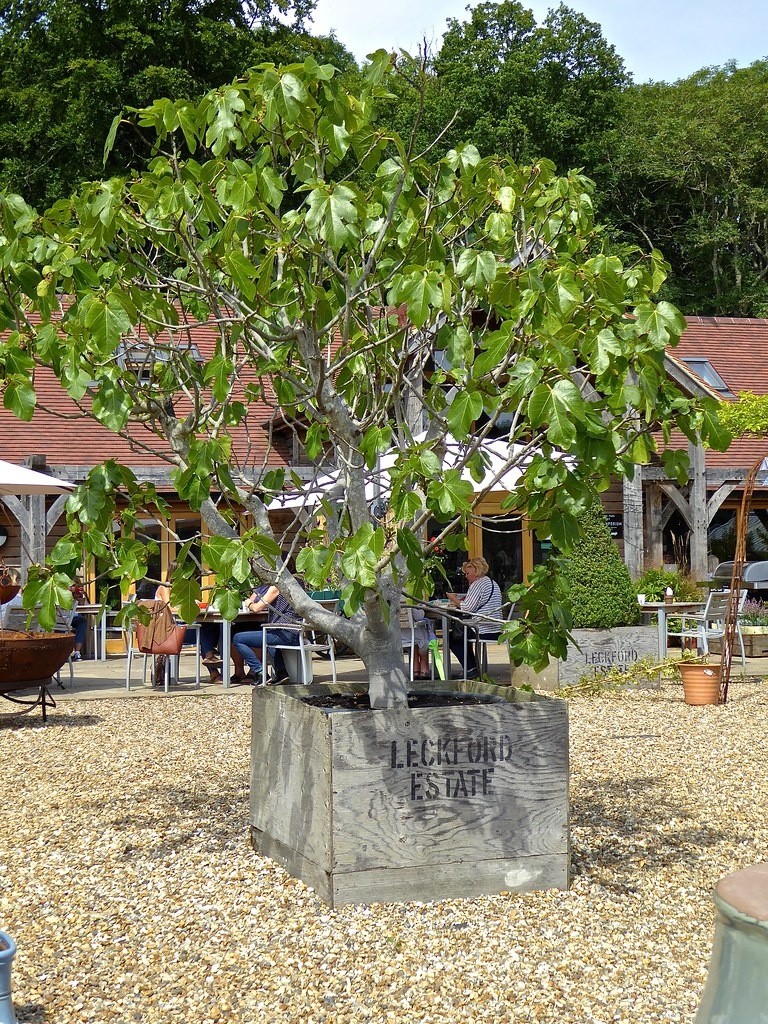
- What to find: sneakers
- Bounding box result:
[270,672,289,686]
[250,674,272,687]
[74,652,83,662]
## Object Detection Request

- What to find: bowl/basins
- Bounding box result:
[198,603,207,608]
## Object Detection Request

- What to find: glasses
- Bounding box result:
[468,559,478,570]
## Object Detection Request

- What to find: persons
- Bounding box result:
[444,556,504,682]
[67,575,90,661]
[398,587,439,680]
[228,551,308,686]
[0,568,44,631]
[155,557,232,685]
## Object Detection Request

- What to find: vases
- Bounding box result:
[677,663,722,705]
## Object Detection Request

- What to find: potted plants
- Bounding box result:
[0,41,731,908]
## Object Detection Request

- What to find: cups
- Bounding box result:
[242,601,249,612]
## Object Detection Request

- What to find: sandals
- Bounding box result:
[230,674,240,684]
[241,674,254,685]
[414,671,420,679]
[420,670,440,680]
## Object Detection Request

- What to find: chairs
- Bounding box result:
[468,602,516,681]
[2,600,79,688]
[94,593,137,663]
[261,600,339,686]
[398,588,435,681]
[667,588,748,669]
[127,599,201,693]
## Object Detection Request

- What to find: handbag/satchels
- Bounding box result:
[453,615,472,639]
[136,601,187,656]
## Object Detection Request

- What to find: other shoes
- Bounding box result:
[208,674,232,684]
[202,657,223,667]
[452,669,477,679]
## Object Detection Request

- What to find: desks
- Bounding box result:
[424,609,467,680]
[75,604,109,661]
[169,611,271,689]
[640,601,706,660]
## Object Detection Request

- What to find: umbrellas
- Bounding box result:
[419,616,446,682]
[0,459,78,498]
[241,427,591,605]
